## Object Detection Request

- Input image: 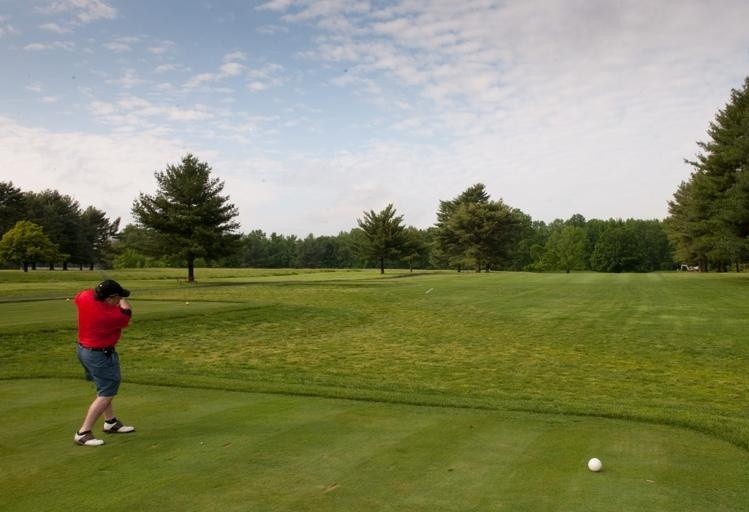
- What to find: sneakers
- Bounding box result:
[102,419,136,435]
[72,431,105,447]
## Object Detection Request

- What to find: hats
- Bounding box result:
[94,279,130,301]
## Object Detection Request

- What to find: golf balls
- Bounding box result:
[586,457,602,474]
[67,299,69,302]
[185,302,189,306]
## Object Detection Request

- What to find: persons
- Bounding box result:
[71,279,136,446]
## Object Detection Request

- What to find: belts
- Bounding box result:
[78,343,110,353]
